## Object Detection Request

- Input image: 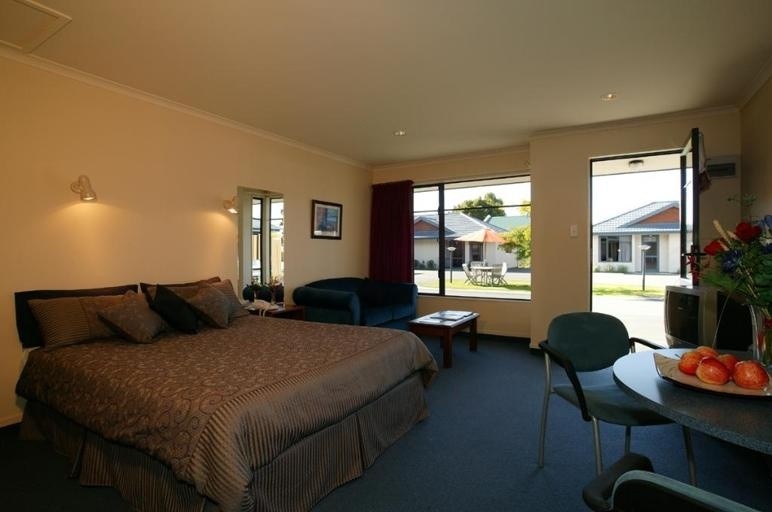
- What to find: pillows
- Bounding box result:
[13,274,250,351]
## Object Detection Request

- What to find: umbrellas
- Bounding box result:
[453,229,505,266]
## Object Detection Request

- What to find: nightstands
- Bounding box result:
[249,302,308,321]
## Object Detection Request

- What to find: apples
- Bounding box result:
[717,353,740,380]
[695,356,728,385]
[696,345,718,357]
[675,352,702,375]
[732,359,769,390]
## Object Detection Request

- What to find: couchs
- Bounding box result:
[291,275,419,334]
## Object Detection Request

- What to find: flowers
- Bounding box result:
[679,190,772,363]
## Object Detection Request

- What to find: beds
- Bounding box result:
[13,273,441,511]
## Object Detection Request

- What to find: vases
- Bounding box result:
[747,301,771,372]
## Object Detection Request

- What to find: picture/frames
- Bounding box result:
[309,198,346,240]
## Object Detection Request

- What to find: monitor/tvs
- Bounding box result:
[665,285,753,352]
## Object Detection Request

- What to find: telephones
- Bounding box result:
[252,299,280,310]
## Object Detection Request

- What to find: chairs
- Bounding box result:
[579,446,771,512]
[462,259,509,288]
[535,310,703,489]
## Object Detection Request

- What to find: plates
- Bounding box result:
[653,352,771,397]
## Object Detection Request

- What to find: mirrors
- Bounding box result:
[236,183,286,304]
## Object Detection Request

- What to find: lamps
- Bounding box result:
[71,174,98,203]
[221,194,241,215]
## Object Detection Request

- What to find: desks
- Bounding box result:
[405,309,480,368]
